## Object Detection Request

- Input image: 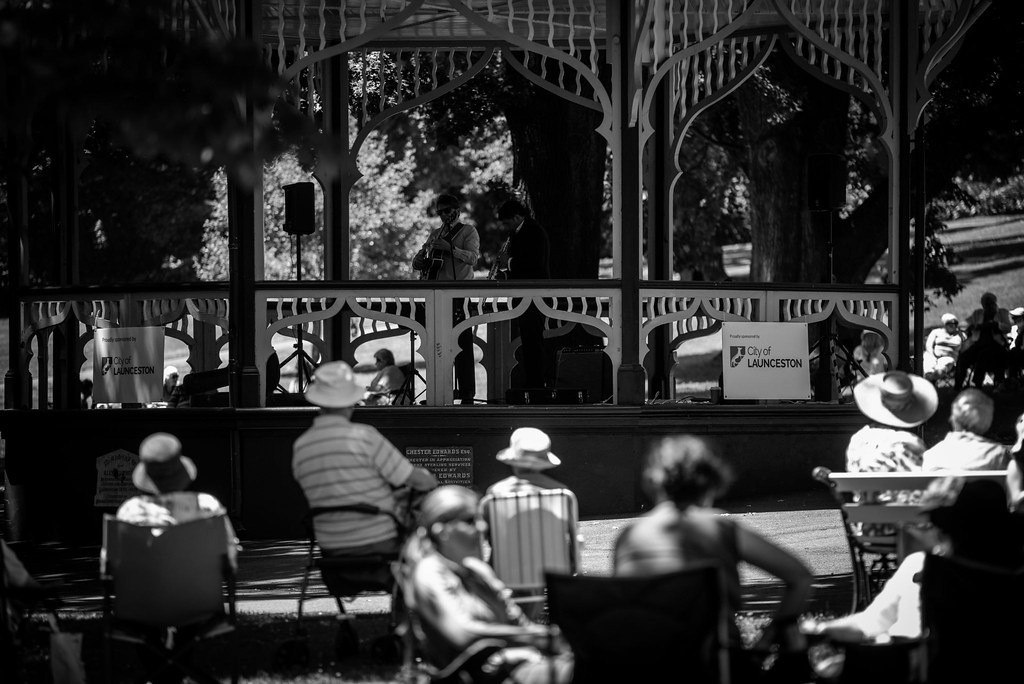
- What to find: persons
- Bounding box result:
[485,426,584,576]
[412,194,551,405]
[802,292,1024,683]
[163,373,179,402]
[80,379,93,409]
[613,433,816,684]
[397,484,574,684]
[98,432,239,580]
[292,361,438,556]
[0,539,36,618]
[366,350,409,408]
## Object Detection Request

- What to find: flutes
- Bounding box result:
[487,231,513,280]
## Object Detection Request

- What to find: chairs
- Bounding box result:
[0,466,1024,684]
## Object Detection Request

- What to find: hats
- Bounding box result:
[1009,307,1024,316]
[853,371,939,428]
[305,360,365,408]
[496,427,561,470]
[133,433,197,493]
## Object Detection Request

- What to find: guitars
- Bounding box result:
[422,208,461,280]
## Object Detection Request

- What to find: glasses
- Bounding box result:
[168,375,178,379]
[436,207,452,215]
